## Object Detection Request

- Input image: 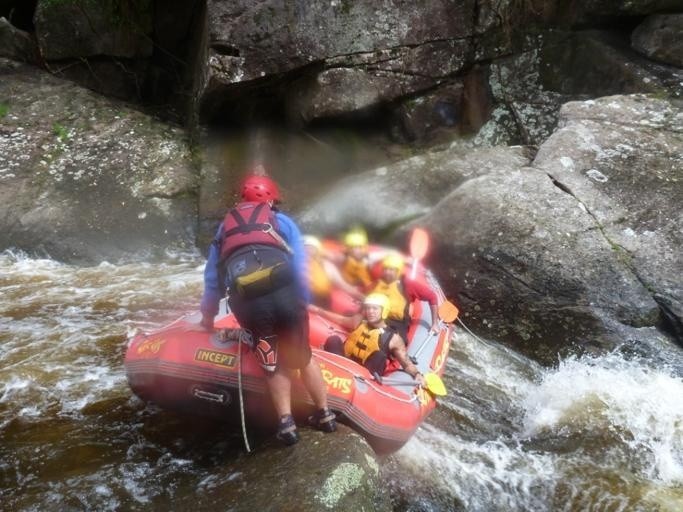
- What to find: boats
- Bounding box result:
[125,243,453,456]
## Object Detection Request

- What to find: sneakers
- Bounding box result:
[303,406,337,432]
[275,414,299,445]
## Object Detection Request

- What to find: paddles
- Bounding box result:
[409,227,428,280]
[373,371,447,396]
[407,300,460,365]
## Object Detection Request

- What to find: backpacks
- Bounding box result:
[215,201,303,338]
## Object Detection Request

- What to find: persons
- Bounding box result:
[323,230,372,293]
[300,232,365,303]
[198,177,338,443]
[365,255,441,343]
[308,293,424,389]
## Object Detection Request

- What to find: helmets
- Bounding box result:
[303,234,321,252]
[363,292,391,320]
[240,175,280,202]
[382,254,403,278]
[344,234,367,247]
